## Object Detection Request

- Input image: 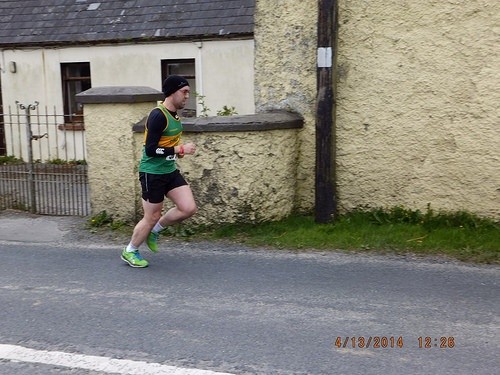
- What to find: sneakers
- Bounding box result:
[120,246,149,268]
[145,232,160,254]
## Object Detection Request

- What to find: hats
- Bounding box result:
[163,75,189,97]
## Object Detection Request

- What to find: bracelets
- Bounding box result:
[179,144,185,154]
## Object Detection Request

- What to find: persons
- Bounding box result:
[118,74,197,269]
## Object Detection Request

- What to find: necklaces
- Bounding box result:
[166,97,179,120]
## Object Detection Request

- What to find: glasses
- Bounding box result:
[178,89,191,94]
